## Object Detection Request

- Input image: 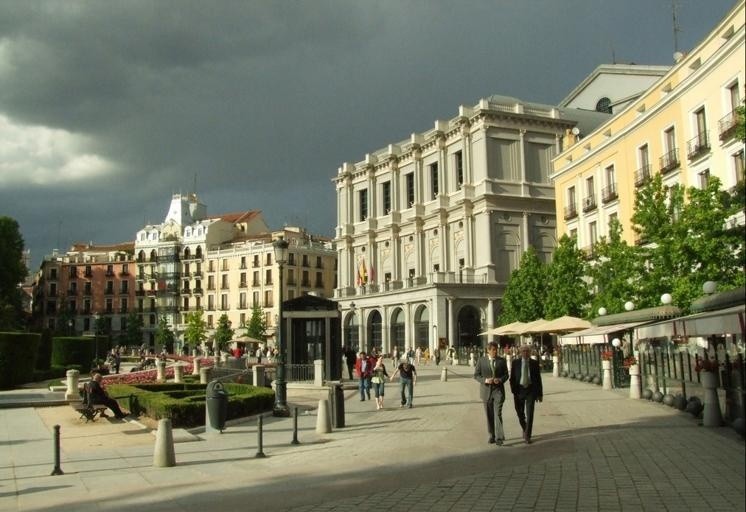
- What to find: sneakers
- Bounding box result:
[100,413,108,417]
[400,403,412,408]
[488,438,503,445]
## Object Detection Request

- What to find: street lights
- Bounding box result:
[350,302,355,351]
[94,312,101,366]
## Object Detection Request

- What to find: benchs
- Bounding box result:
[69,383,107,424]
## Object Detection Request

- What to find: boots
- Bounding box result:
[376,396,384,410]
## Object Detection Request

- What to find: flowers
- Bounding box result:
[694,354,727,374]
[601,350,637,368]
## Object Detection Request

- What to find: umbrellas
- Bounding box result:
[225,335,262,343]
[525,314,592,333]
[476,320,524,336]
[499,317,549,345]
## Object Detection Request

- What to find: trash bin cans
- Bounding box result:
[204,379,230,433]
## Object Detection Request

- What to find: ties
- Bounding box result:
[523,360,528,389]
[491,359,495,378]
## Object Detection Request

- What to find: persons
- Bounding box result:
[89,344,168,375]
[390,353,417,409]
[229,346,278,364]
[345,347,356,381]
[509,345,542,445]
[86,373,128,418]
[339,347,350,382]
[366,355,391,411]
[367,345,489,367]
[472,341,509,447]
[354,352,375,401]
